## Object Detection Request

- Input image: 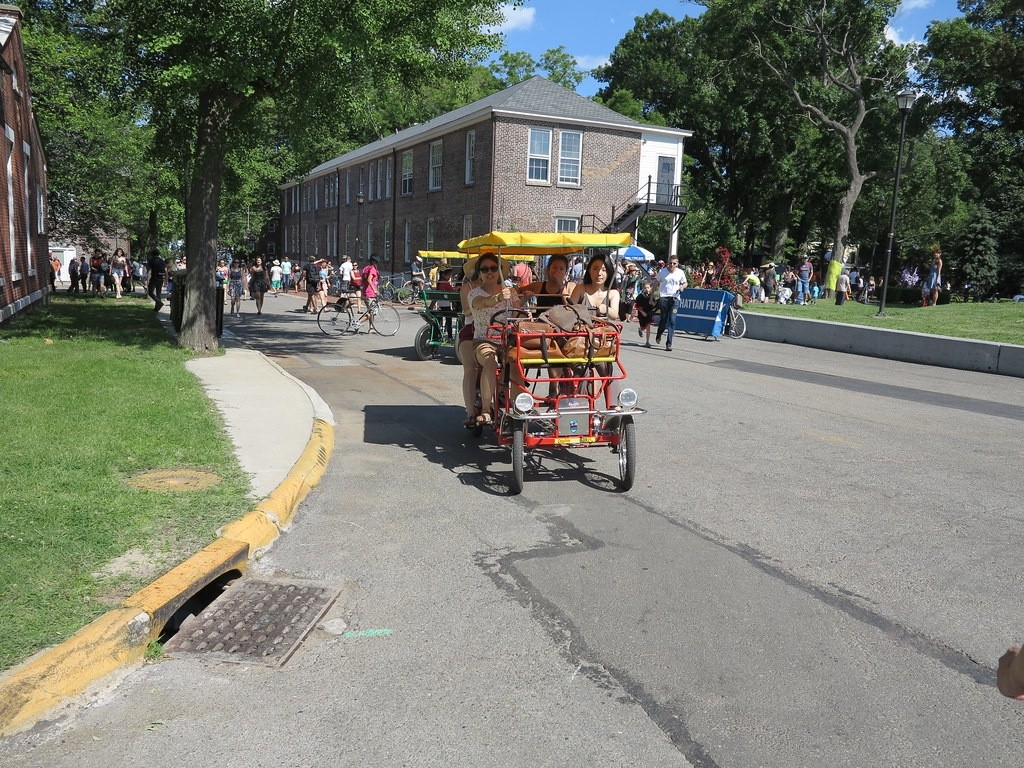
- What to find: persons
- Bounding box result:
[514,261,532,311]
[520,253,577,397]
[700,262,716,288]
[835,267,875,305]
[456,252,520,427]
[921,250,942,307]
[217,256,304,318]
[529,261,539,309]
[410,256,460,343]
[49,248,187,313]
[569,255,620,377]
[944,281,951,291]
[878,276,883,286]
[650,255,688,351]
[303,254,380,333]
[763,254,819,305]
[615,260,665,348]
[739,267,761,301]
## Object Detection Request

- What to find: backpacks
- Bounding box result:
[306,263,320,282]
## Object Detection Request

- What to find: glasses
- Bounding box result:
[353,265,357,266]
[234,262,239,263]
[480,266,498,273]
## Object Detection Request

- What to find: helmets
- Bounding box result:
[438,263,453,273]
[416,256,423,262]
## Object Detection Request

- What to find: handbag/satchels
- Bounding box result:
[506,303,618,362]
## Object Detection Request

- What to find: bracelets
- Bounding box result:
[495,295,499,303]
[559,287,564,291]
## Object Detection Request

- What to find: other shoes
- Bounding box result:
[639,330,643,337]
[236,314,240,317]
[258,311,261,315]
[368,330,380,334]
[155,302,164,312]
[666,346,672,350]
[656,334,661,344]
[646,343,650,347]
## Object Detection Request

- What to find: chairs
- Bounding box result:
[421,290,462,340]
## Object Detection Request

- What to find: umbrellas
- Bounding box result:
[611,245,654,261]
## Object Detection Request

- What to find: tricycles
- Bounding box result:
[415,230,647,494]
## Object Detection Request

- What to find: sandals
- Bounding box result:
[479,411,491,424]
[463,416,475,425]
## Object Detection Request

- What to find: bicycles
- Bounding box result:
[684,294,747,339]
[377,272,420,305]
[317,291,400,336]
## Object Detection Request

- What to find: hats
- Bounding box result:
[770,263,777,267]
[463,253,510,284]
[803,255,809,259]
[273,260,280,265]
[307,256,315,261]
[627,265,639,273]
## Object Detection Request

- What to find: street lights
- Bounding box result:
[355,192,364,249]
[875,90,916,318]
